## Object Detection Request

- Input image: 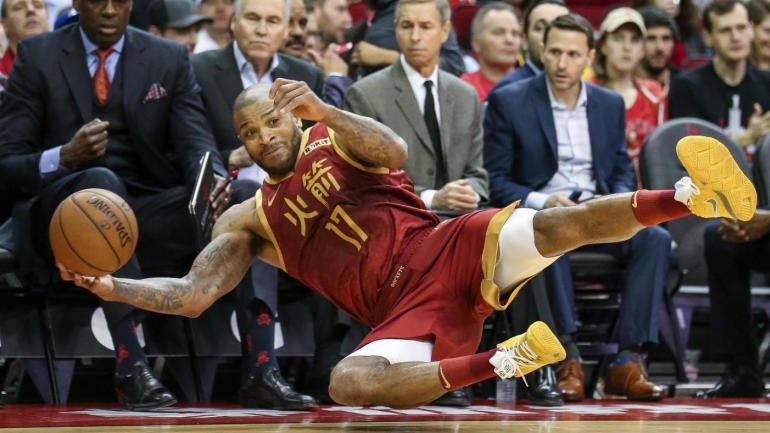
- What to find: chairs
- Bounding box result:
[0,111,770,410]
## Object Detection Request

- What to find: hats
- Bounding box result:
[601,8,647,36]
[130,0,215,30]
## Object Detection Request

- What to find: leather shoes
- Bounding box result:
[695,371,764,397]
[556,361,585,400]
[114,362,177,411]
[519,366,564,406]
[236,363,316,409]
[604,360,663,399]
[437,389,471,406]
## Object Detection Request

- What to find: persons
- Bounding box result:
[0,0,770,410]
[55,75,757,410]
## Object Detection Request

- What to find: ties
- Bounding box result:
[423,80,448,190]
[94,45,113,105]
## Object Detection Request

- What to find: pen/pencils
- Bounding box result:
[216,169,238,197]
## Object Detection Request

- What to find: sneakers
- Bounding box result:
[676,136,757,221]
[489,321,567,387]
